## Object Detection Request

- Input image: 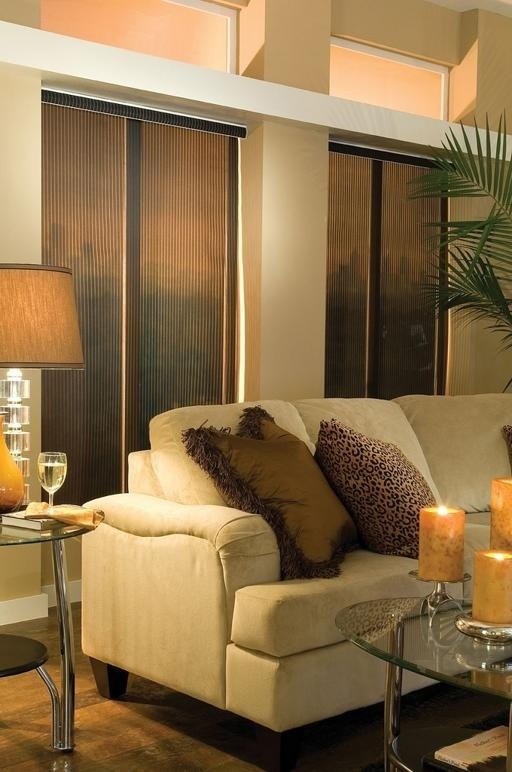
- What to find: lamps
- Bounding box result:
[0,264,87,507]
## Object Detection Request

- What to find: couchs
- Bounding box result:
[83,392,511,769]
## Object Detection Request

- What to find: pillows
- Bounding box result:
[314,417,436,560]
[181,405,359,578]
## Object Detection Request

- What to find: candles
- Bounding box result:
[410,475,510,628]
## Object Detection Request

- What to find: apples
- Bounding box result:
[410,475,510,628]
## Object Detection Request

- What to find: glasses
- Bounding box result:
[0,264,87,507]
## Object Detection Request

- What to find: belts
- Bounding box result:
[36,452,67,516]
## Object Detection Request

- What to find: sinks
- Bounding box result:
[83,392,511,769]
